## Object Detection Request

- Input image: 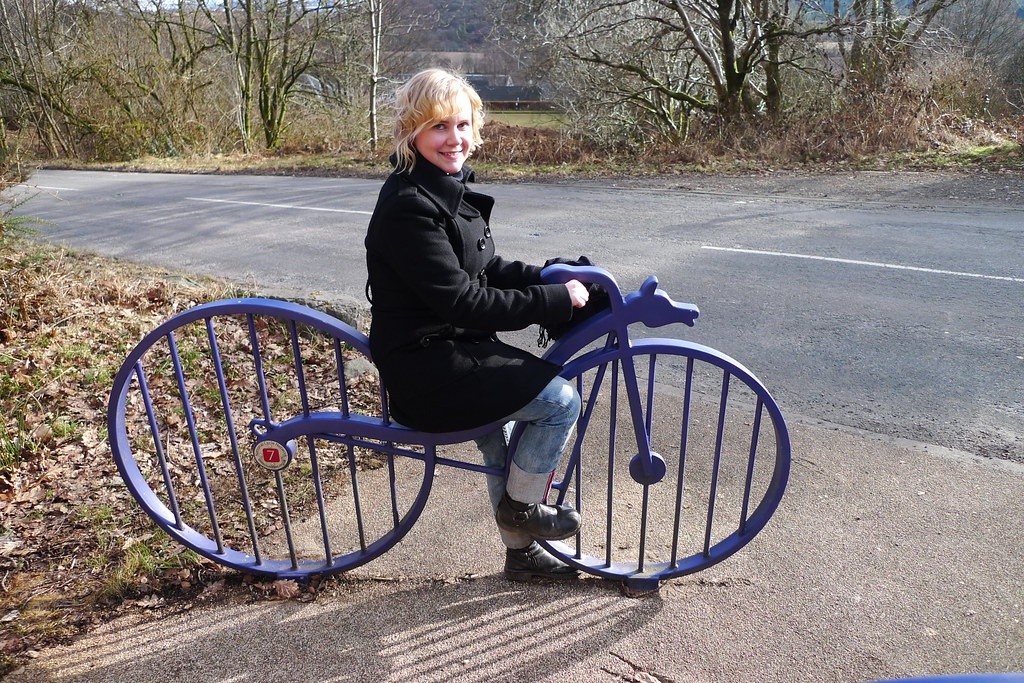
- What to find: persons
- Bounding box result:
[364,68,589,578]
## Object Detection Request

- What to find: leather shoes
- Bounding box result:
[504,540,582,580]
[496,491,581,540]
